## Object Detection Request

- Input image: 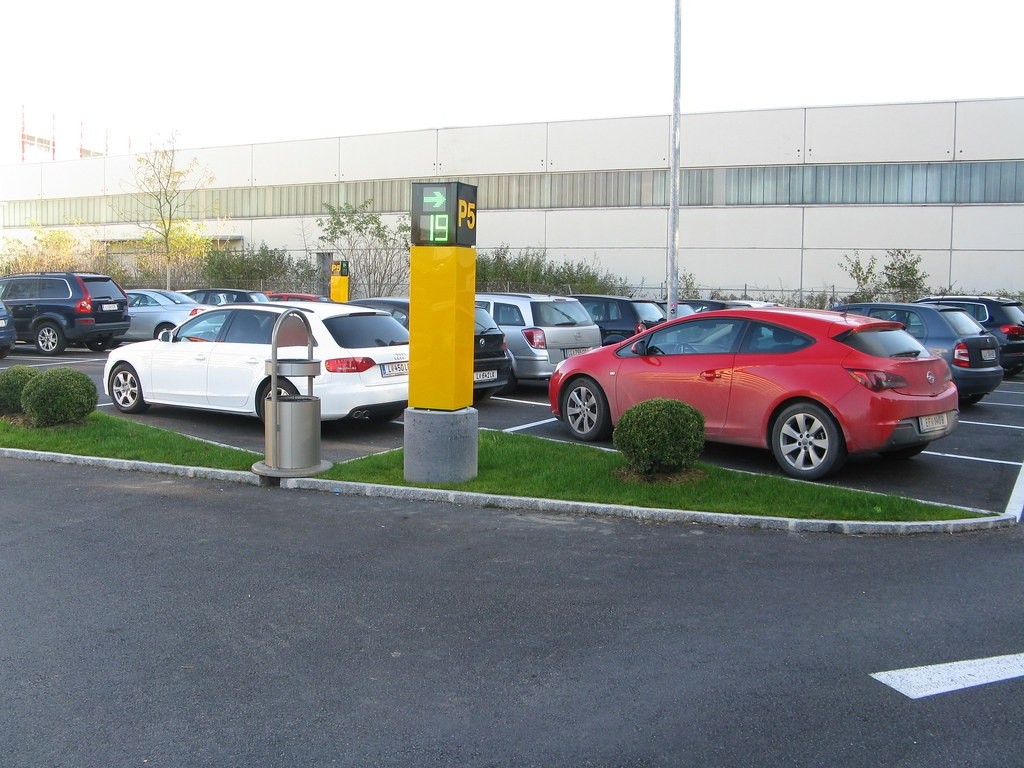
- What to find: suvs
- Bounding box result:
[476,290,601,392]
[913,295,1024,381]
[0,270,132,356]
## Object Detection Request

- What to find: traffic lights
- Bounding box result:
[420,186,450,242]
[343,263,348,276]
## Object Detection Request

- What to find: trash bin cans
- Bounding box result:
[250,309,334,477]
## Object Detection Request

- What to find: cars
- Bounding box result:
[548,307,960,482]
[346,296,513,406]
[102,302,409,431]
[681,298,784,315]
[113,288,214,342]
[566,293,666,347]
[0,299,16,361]
[185,288,272,307]
[829,303,1004,406]
[267,293,330,302]
[656,299,697,319]
